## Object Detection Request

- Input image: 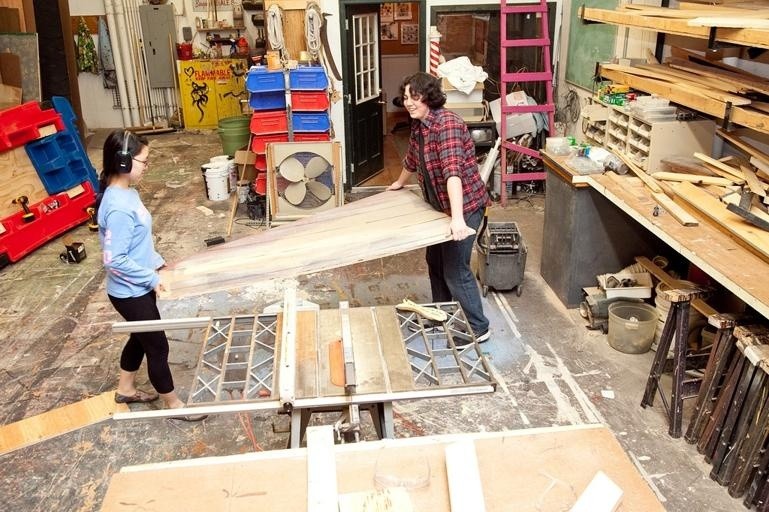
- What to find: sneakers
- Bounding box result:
[114,389,160,404]
[446,327,490,350]
[407,311,455,334]
[165,414,208,422]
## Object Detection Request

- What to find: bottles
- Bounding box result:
[196,17,227,29]
[205,42,222,59]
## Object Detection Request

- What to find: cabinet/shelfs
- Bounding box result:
[198,26,247,45]
[581,94,717,178]
[577,0,769,139]
[175,58,250,132]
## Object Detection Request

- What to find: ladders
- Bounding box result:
[500,0,556,209]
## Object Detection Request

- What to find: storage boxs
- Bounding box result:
[488,90,535,140]
[234,144,257,182]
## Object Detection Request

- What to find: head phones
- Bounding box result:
[116,130,132,173]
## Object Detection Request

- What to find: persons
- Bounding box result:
[385,72,492,350]
[229,37,252,70]
[92,129,208,422]
[247,48,265,66]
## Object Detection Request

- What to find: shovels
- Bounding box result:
[255,28,266,49]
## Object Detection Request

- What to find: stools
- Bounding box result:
[391,96,412,135]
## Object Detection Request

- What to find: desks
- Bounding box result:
[539,146,769,326]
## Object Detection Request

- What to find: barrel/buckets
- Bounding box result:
[217,115,251,156]
[606,300,659,355]
[265,49,283,70]
[200,163,232,203]
[236,179,250,205]
[176,43,193,61]
[209,155,238,193]
[649,279,711,360]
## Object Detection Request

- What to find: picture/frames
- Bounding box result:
[192,0,242,12]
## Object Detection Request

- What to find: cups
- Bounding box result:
[264,51,281,70]
